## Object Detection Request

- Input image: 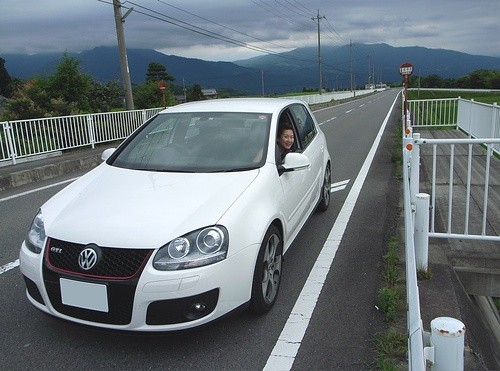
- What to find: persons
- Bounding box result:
[253,123,294,162]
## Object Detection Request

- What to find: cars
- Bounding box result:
[18,97,332,336]
